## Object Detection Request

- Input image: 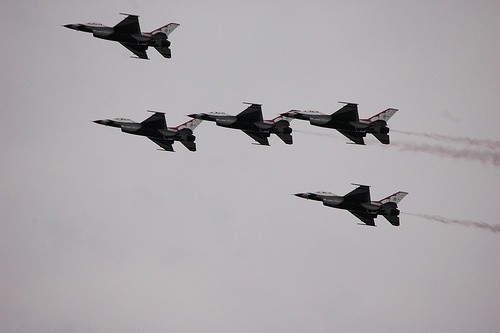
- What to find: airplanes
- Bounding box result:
[186,102,301,146]
[278,102,398,145]
[293,183,409,227]
[91,110,203,152]
[60,13,180,60]
